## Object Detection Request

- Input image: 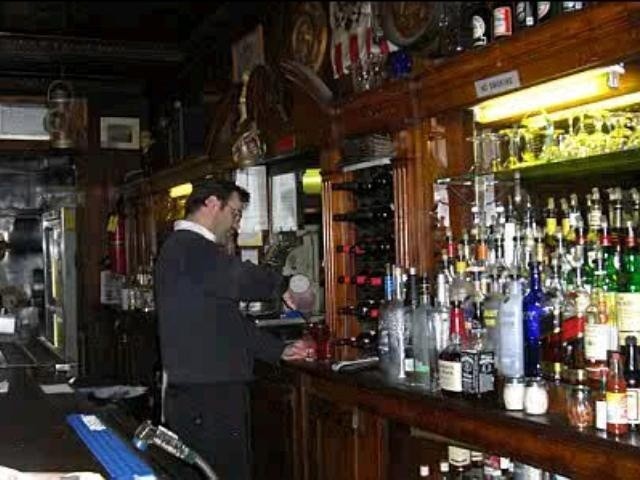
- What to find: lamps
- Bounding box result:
[46,81,87,150]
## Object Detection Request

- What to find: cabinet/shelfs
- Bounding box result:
[321,109,413,372]
[384,0,639,480]
[83,313,162,386]
[242,359,385,480]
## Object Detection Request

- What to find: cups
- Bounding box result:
[288,271,317,313]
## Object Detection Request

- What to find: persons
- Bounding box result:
[155,179,310,480]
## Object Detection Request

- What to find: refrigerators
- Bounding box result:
[39,203,77,363]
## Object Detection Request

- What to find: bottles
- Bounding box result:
[442,172,640,290]
[379,258,640,441]
[328,170,399,358]
[468,0,586,49]
[414,445,562,480]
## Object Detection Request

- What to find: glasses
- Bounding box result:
[221,201,243,225]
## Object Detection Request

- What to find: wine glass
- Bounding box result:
[466,106,639,173]
